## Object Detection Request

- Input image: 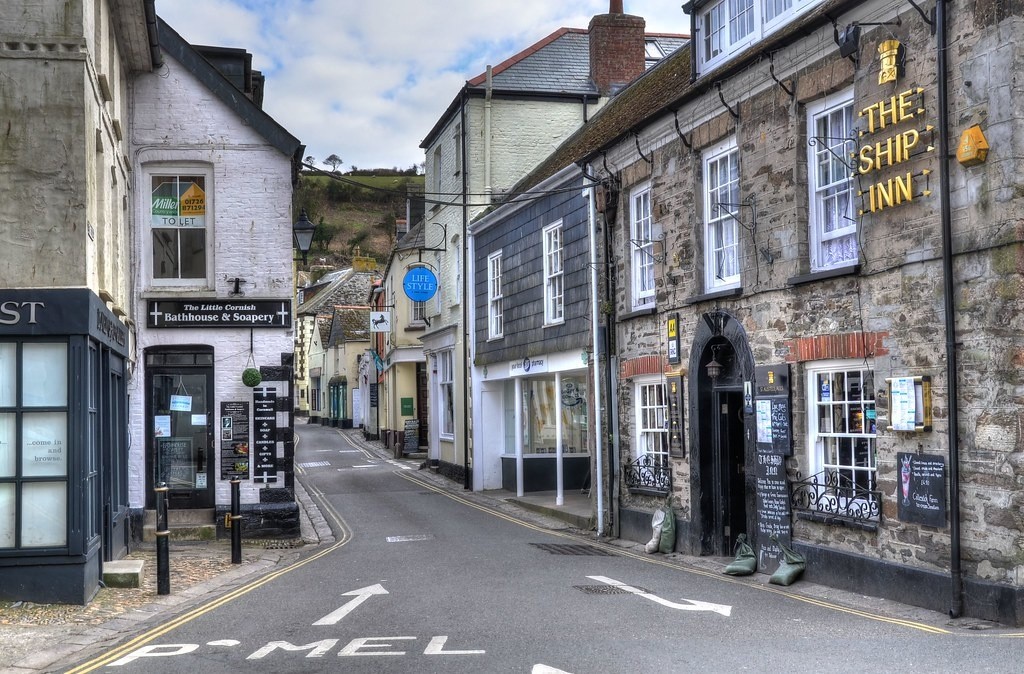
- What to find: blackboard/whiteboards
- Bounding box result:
[403,419,419,453]
[755,394,791,455]
[157,437,196,490]
[896,452,947,529]
[755,453,792,575]
